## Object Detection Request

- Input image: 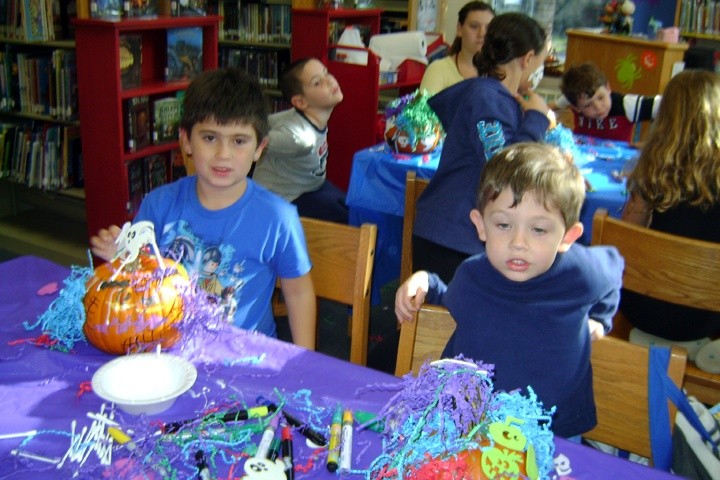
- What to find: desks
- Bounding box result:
[347,136,642,308]
[0,254,691,480]
[562,27,690,129]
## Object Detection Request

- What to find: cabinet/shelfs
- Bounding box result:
[0,0,448,273]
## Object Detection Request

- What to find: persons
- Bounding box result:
[617,66,720,376]
[360,12,550,374]
[250,56,349,227]
[417,0,498,100]
[394,139,625,443]
[547,60,663,145]
[88,64,320,351]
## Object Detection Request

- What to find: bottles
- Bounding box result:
[337,26,364,60]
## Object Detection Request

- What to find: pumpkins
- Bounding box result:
[82,246,189,354]
[384,91,441,154]
[428,433,528,480]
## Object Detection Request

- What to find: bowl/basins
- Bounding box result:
[91,353,198,416]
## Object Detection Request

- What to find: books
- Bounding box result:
[679,0,720,36]
[0,0,290,213]
[316,0,408,47]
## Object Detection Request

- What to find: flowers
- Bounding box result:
[601,1,636,38]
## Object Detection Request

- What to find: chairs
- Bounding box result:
[590,210,720,407]
[272,219,377,371]
[394,296,687,471]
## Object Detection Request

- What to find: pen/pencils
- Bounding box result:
[282,427,297,480]
[326,411,343,472]
[106,427,169,477]
[340,410,353,472]
[160,406,268,434]
[243,414,279,479]
[266,437,280,465]
[256,395,328,447]
